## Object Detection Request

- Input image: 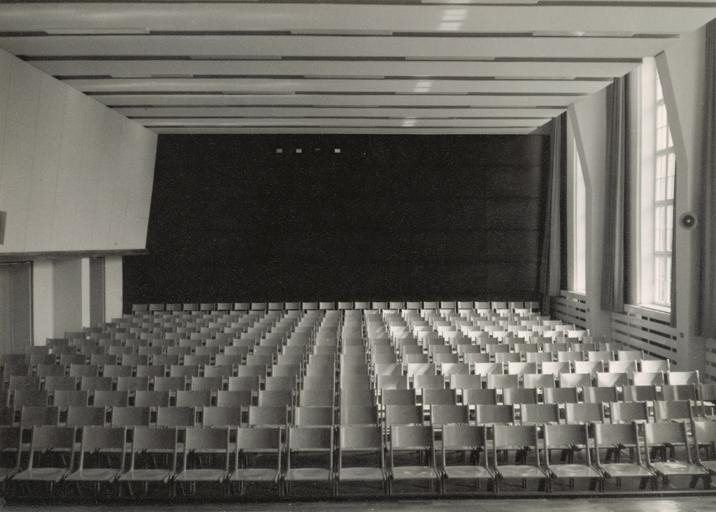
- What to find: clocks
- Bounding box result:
[679,212,696,232]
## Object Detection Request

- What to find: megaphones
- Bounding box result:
[679,210,698,230]
